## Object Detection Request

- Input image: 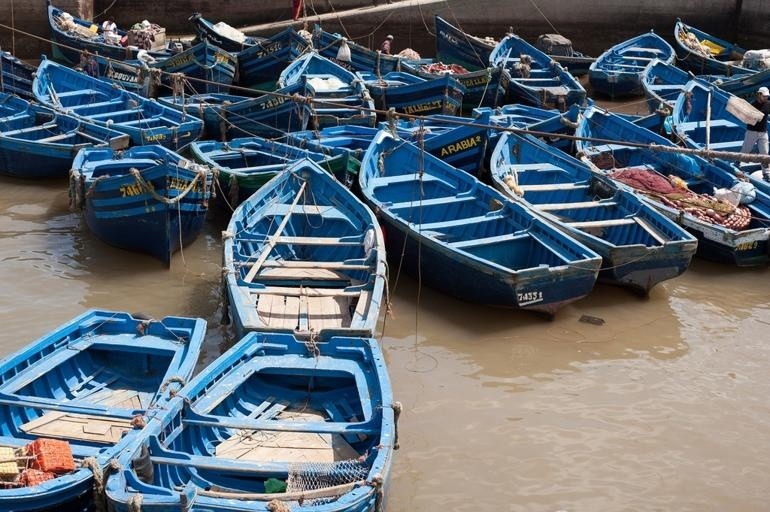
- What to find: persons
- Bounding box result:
[734,87,770,181]
[102,16,117,44]
[380,34,394,54]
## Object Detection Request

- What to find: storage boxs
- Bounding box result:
[128,28,167,52]
[725,96,765,125]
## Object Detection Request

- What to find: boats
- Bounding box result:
[0,308,208,511]
[104,330,394,512]
